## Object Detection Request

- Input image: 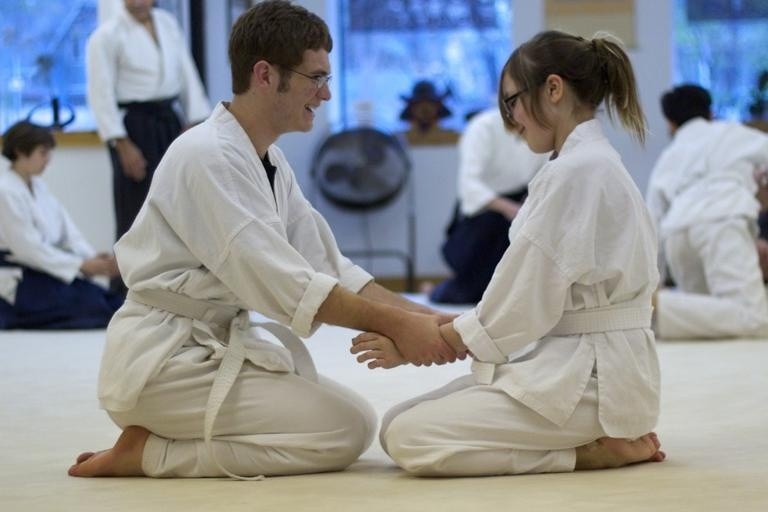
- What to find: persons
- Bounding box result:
[59,0,461,483]
[0,117,127,333]
[643,80,767,342]
[416,100,554,307]
[343,25,670,484]
[391,79,461,147]
[82,0,213,246]
[753,210,767,284]
[743,70,767,122]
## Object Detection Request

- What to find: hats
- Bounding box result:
[397,80,454,124]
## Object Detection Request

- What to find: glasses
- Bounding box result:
[502,86,533,115]
[281,67,334,87]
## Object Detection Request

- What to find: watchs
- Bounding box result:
[108,137,126,149]
